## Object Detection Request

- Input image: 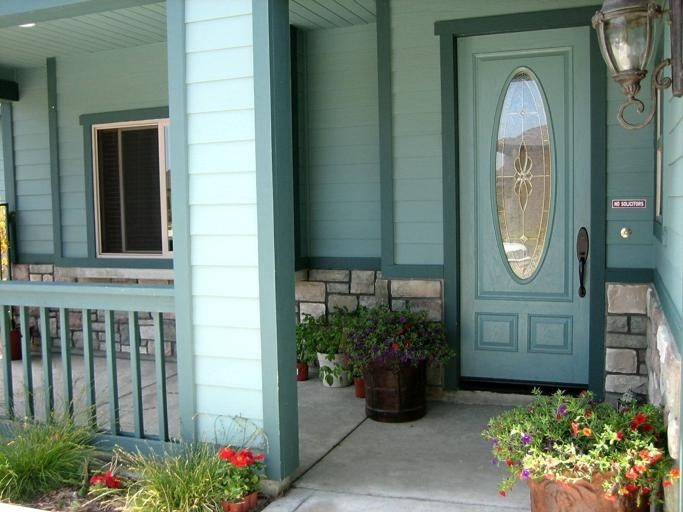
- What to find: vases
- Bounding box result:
[221,491,259,511]
[364,364,427,424]
[9,329,23,360]
[527,471,650,512]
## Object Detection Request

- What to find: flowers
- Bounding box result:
[218,445,266,501]
[478,386,681,506]
[347,317,438,364]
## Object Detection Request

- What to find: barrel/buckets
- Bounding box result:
[316,350,427,425]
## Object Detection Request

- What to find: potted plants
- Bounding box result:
[296,303,363,400]
